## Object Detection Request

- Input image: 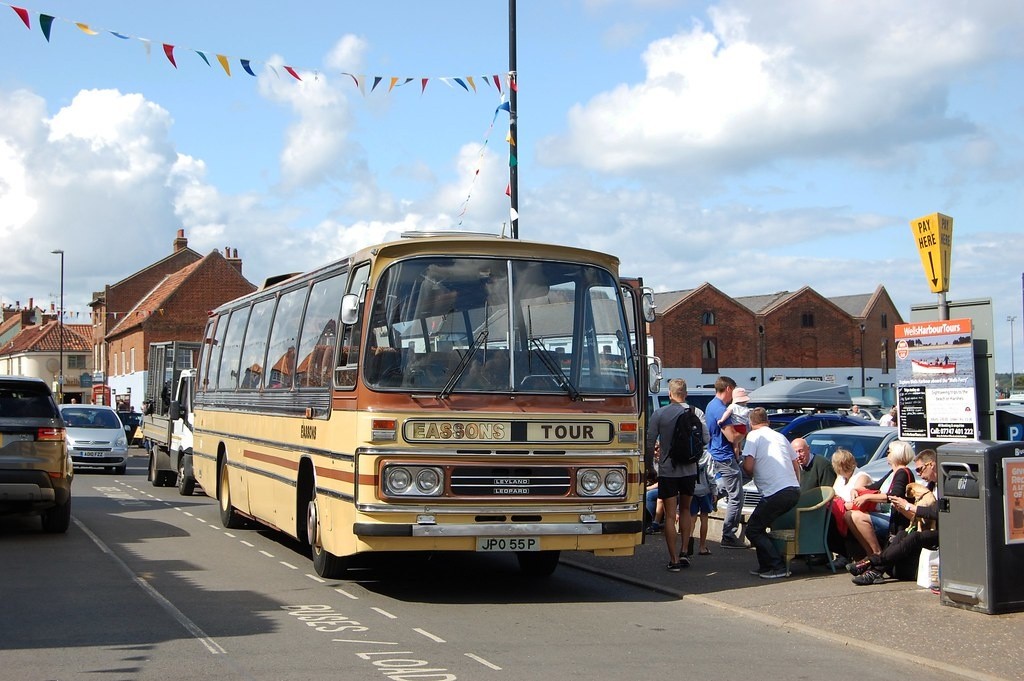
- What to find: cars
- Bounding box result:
[118,410,142,448]
[56,403,132,476]
[643,379,1024,517]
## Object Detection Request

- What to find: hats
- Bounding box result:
[732,387,750,403]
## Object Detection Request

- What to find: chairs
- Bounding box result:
[767,486,836,578]
[249,346,561,388]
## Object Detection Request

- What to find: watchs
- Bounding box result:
[905,503,910,511]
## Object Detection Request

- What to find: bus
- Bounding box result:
[193,230,662,578]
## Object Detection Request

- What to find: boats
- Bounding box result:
[911,358,957,375]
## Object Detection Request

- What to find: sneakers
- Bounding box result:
[759,569,788,578]
[851,568,883,585]
[845,555,871,575]
[827,554,854,570]
[720,538,747,549]
[749,567,770,576]
[806,555,830,565]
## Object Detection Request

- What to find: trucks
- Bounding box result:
[140,367,197,495]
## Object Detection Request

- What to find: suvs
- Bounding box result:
[0,374,75,534]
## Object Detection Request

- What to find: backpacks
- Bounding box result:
[668,405,703,463]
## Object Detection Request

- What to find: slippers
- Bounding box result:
[699,548,712,555]
[687,536,695,556]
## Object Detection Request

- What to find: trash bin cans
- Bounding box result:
[936,442,1024,615]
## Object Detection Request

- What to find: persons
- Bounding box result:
[812,405,865,417]
[139,401,154,456]
[920,352,950,365]
[999,392,1005,398]
[846,449,938,585]
[72,398,76,404]
[826,449,881,569]
[117,399,127,410]
[844,440,915,555]
[90,399,94,405]
[743,406,837,578]
[77,409,92,424]
[130,406,138,419]
[646,376,754,571]
[879,406,897,426]
[91,415,104,426]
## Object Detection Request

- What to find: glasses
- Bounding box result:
[915,461,932,473]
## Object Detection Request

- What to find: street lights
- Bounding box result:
[49,249,64,406]
[1006,314,1017,394]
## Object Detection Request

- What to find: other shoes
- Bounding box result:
[649,522,660,532]
[666,560,681,572]
[679,553,690,567]
